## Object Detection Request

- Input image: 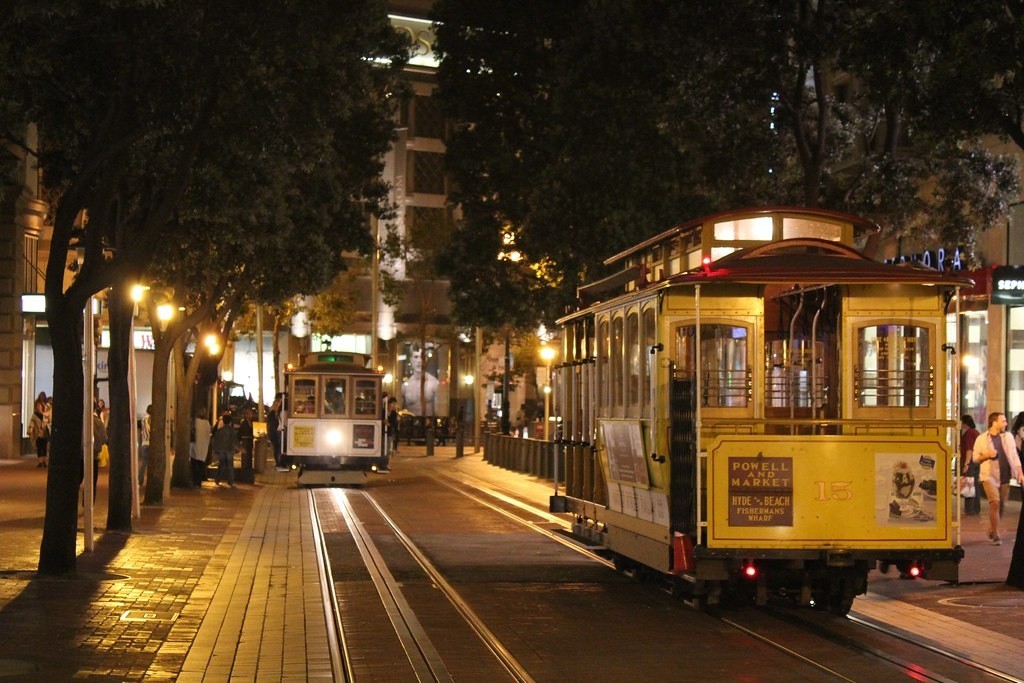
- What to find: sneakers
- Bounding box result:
[990,533,1003,545]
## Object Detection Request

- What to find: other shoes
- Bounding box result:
[215,481,236,488]
[37,462,47,468]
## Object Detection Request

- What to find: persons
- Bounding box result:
[401,343,441,417]
[1011,411,1024,456]
[27,390,495,489]
[972,412,1024,545]
[515,404,532,437]
[954,414,982,515]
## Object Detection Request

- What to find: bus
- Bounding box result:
[548,204,967,628]
[278,346,387,489]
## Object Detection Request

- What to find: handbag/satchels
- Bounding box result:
[952,472,976,498]
[46,422,51,440]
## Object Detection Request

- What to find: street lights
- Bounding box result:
[79,276,228,561]
